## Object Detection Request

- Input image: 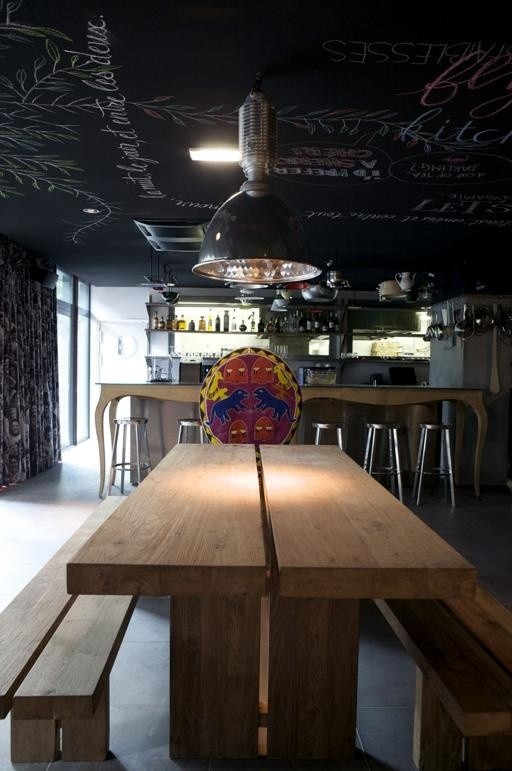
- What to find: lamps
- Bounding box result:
[192,72,322,284]
[143,242,181,307]
[270,284,288,313]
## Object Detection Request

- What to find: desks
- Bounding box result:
[93,384,489,498]
[67,442,479,760]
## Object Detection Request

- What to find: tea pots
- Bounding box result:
[394,272,417,291]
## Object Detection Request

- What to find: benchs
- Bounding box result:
[0,495,140,761]
[372,586,512,770]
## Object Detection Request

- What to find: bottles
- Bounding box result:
[171,350,217,363]
[152,311,339,333]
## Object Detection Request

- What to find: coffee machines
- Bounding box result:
[145,356,172,383]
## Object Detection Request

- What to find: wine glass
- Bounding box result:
[273,343,289,359]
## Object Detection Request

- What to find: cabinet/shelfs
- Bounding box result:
[143,300,434,366]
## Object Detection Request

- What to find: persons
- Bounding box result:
[0,234,61,491]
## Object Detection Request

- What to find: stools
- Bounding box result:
[177,417,206,443]
[364,420,404,505]
[106,418,151,495]
[412,422,458,507]
[312,420,345,451]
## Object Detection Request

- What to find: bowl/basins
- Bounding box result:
[280,282,338,302]
